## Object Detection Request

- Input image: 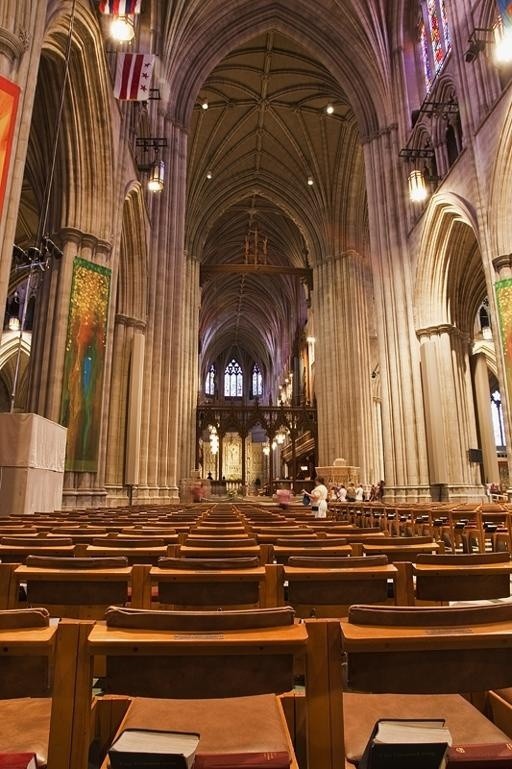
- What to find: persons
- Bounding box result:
[275,482,292,502]
[300,476,328,518]
[329,479,385,502]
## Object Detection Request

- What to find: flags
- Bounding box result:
[111,52,156,101]
[96,1,143,19]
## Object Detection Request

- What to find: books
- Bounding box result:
[0,752,38,768]
[358,717,453,769]
[105,728,201,769]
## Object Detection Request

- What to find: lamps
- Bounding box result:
[13,236,64,272]
[111,12,136,41]
[136,137,168,191]
[408,170,443,202]
[466,41,485,63]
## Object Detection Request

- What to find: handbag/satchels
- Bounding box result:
[312,506,318,511]
[303,494,311,506]
